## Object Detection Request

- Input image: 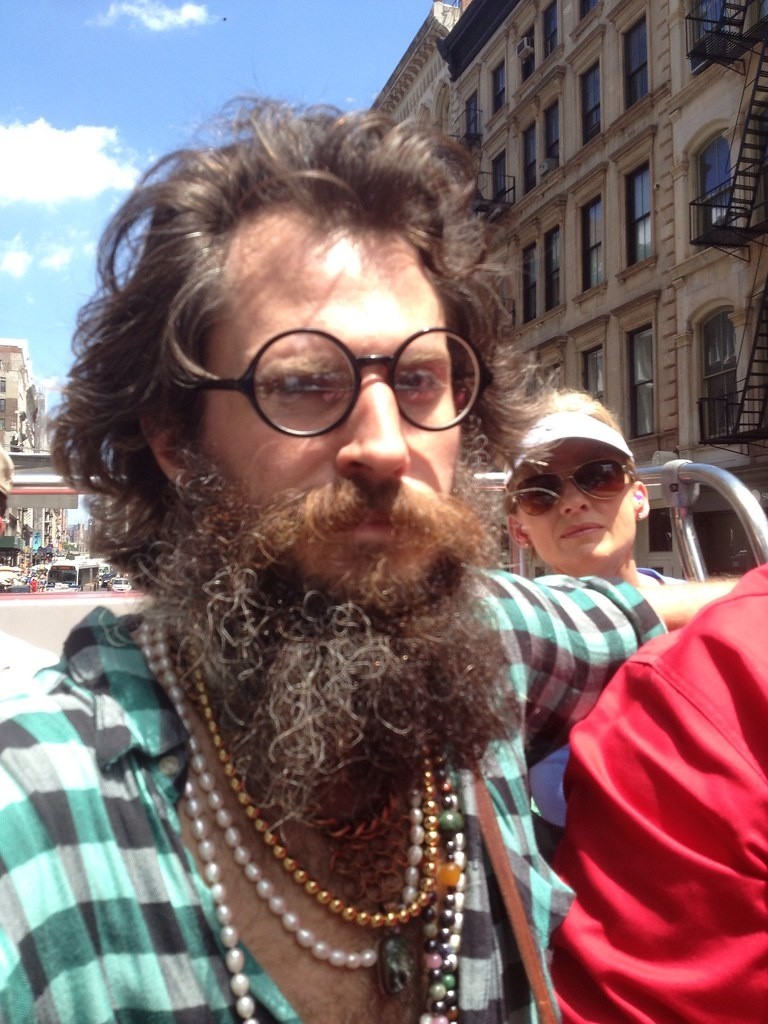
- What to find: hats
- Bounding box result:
[502,413,635,495]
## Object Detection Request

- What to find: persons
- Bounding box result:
[0,97,742,1024]
[548,566,768,1024]
[503,390,695,833]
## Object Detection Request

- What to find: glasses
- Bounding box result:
[149,328,481,437]
[512,460,632,516]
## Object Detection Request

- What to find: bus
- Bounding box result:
[45,560,100,593]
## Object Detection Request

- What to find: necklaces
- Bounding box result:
[133,607,469,1024]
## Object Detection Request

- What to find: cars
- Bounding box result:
[99,567,132,594]
